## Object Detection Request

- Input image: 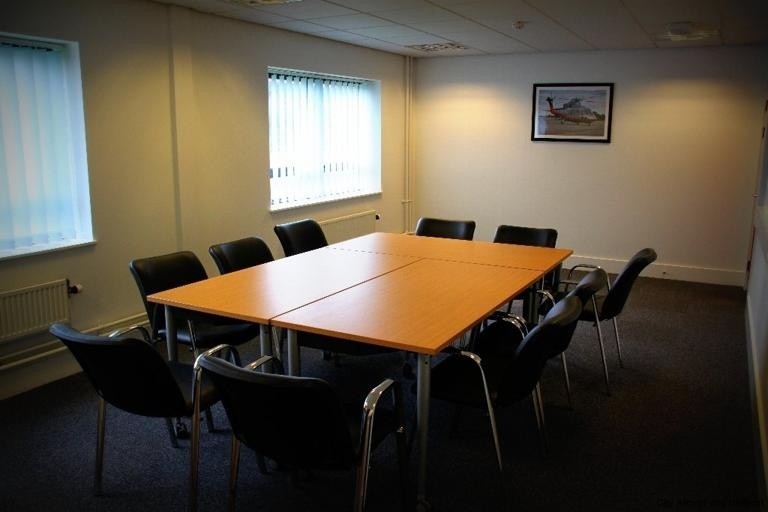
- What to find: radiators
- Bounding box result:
[0,278,83,346]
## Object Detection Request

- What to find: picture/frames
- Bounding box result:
[530,82,615,144]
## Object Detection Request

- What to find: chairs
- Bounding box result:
[537,248,657,398]
[415,216,476,241]
[47,323,244,512]
[198,354,408,512]
[128,218,329,371]
[465,288,584,441]
[493,225,559,314]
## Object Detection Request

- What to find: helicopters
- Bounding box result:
[540,95,600,127]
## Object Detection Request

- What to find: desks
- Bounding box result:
[328,232,574,323]
[146,230,421,474]
[270,256,543,512]
